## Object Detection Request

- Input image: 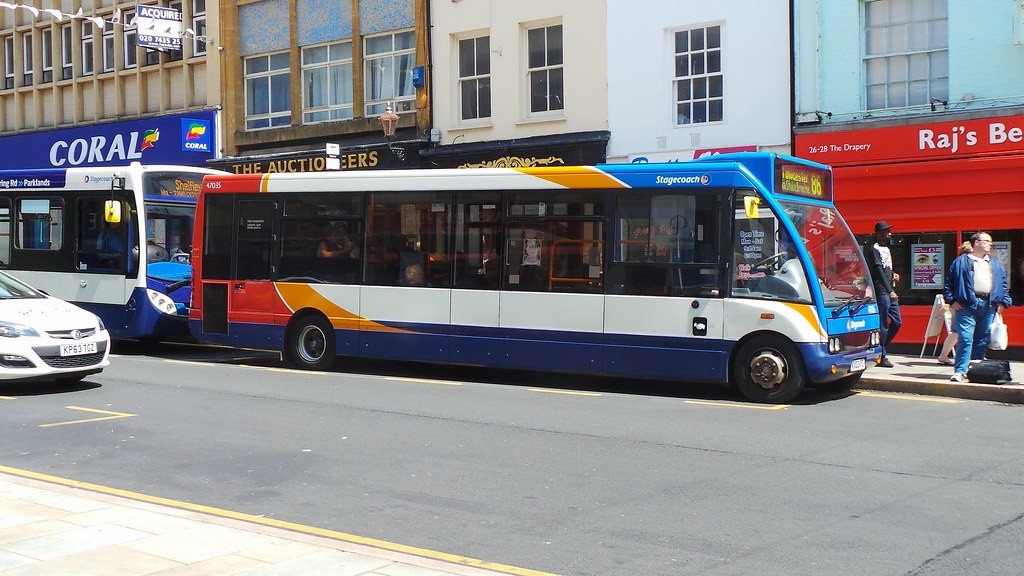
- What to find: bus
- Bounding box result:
[0,161,236,347]
[187,151,885,404]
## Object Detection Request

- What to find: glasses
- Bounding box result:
[978,240,993,244]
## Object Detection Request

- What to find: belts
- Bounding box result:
[975,293,989,298]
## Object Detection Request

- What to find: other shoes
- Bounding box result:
[951,370,963,382]
[881,356,892,367]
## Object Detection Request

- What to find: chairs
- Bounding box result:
[96,215,104,250]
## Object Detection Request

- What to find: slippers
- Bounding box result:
[936,362,955,366]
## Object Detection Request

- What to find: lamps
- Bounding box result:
[930,97,947,112]
[376,99,407,162]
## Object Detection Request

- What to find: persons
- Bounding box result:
[914,253,938,264]
[317,222,360,257]
[866,221,902,367]
[405,265,424,285]
[943,232,1012,382]
[96,222,123,269]
[937,241,973,366]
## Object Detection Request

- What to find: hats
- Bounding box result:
[875,221,892,232]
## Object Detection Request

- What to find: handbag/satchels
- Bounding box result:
[988,312,1008,350]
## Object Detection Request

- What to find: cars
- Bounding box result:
[0,270,110,385]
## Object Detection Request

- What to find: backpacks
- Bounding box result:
[967,359,1012,385]
